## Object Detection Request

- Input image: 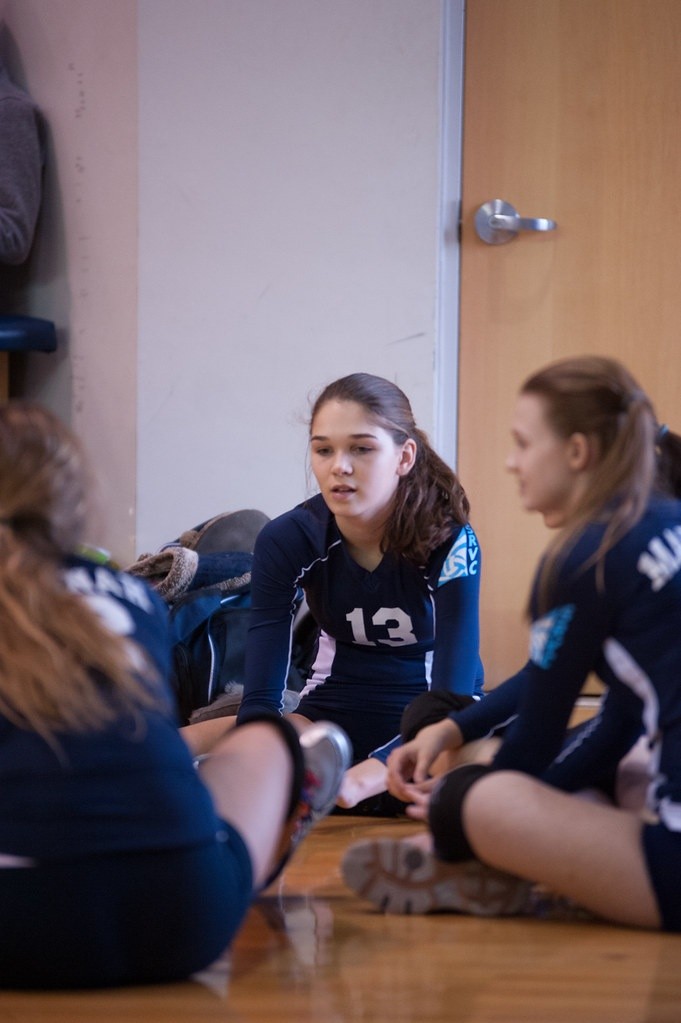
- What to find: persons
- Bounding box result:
[339,355,681,932]
[159,372,487,817]
[0,400,351,993]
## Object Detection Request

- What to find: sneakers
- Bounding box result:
[338,833,536,918]
[262,723,351,886]
